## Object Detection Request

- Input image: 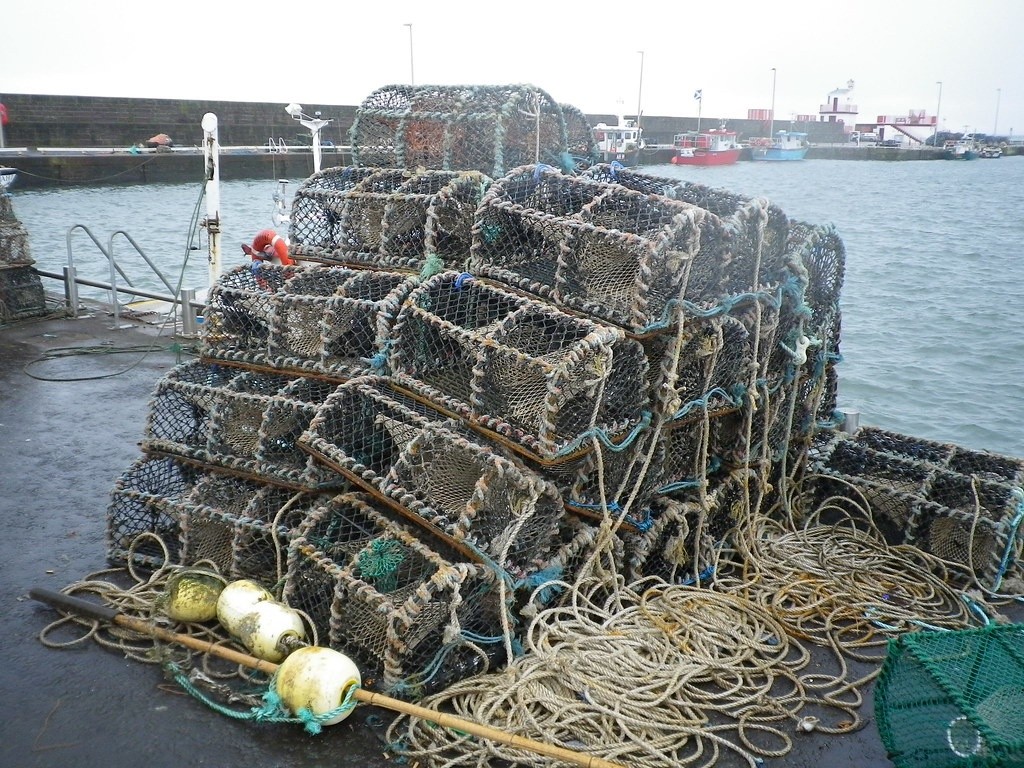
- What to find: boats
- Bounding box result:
[672,90,743,166]
[145,133,174,153]
[937,125,1003,161]
[746,129,810,160]
[592,100,646,167]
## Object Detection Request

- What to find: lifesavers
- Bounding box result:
[251,230,294,293]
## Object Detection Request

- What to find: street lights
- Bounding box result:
[637,51,644,127]
[404,24,414,85]
[994,89,1001,136]
[771,68,776,138]
[934,82,942,146]
